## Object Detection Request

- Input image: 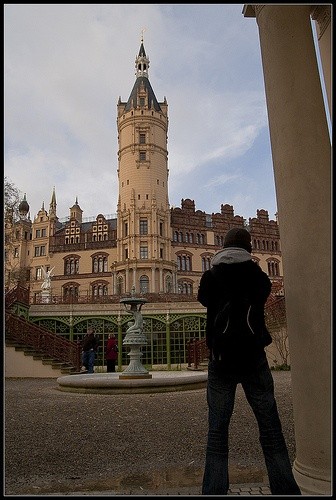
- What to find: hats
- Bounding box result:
[223,228,253,252]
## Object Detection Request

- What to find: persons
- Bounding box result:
[124,302,144,333]
[40,266,55,289]
[195,227,302,494]
[80,326,100,372]
[105,333,119,373]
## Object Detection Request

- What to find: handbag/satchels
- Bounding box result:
[110,344,118,351]
